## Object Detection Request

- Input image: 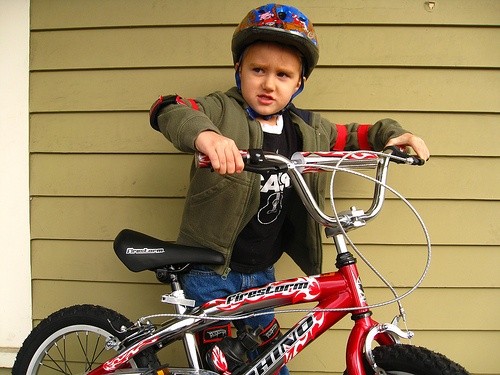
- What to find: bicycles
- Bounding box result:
[12,146,472,375]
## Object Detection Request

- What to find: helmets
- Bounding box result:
[232,3,320,81]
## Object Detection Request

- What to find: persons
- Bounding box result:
[149,4,431,375]
